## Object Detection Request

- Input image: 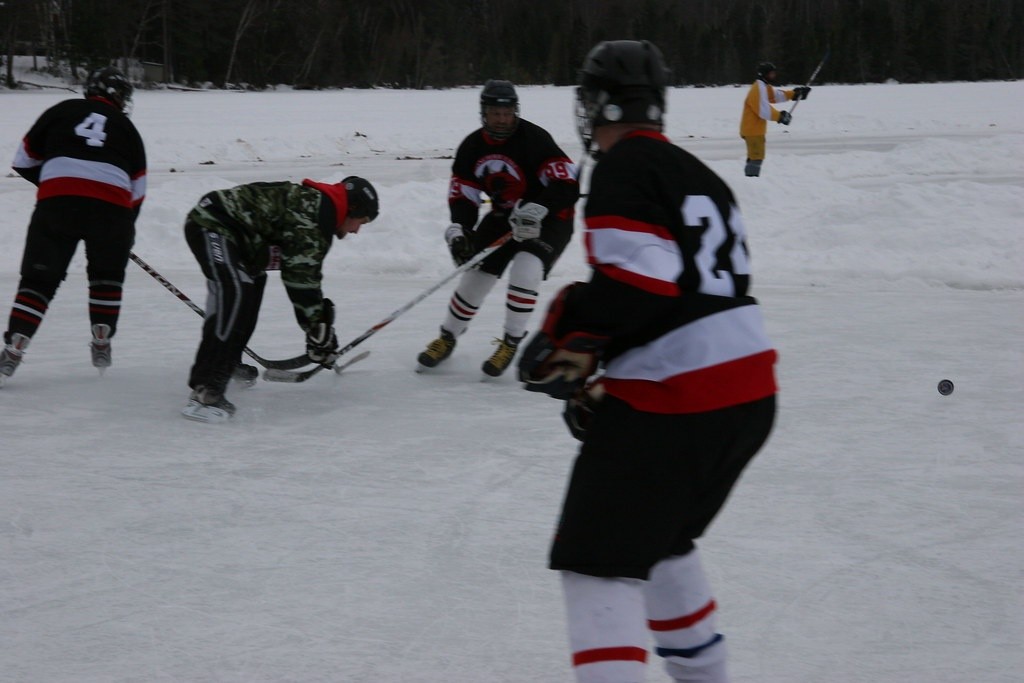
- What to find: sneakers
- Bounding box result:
[415,324,466,374]
[0,331,30,387]
[182,384,235,422]
[481,331,528,381]
[226,363,258,388]
[91,324,111,375]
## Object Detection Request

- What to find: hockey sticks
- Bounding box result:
[789,41,832,114]
[260,231,515,384]
[330,350,372,375]
[127,250,315,370]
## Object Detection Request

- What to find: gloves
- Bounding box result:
[445,223,483,271]
[519,282,610,398]
[792,87,810,100]
[563,369,607,442]
[306,323,337,370]
[508,198,550,250]
[778,110,791,125]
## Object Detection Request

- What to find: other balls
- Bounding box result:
[937,379,955,395]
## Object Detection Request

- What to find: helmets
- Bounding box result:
[341,176,378,221]
[573,40,664,159]
[84,65,132,114]
[757,62,776,80]
[480,79,519,140]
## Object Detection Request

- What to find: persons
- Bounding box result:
[0,66,147,376]
[514,39,776,683]
[740,61,810,176]
[185,176,379,421]
[418,78,579,376]
